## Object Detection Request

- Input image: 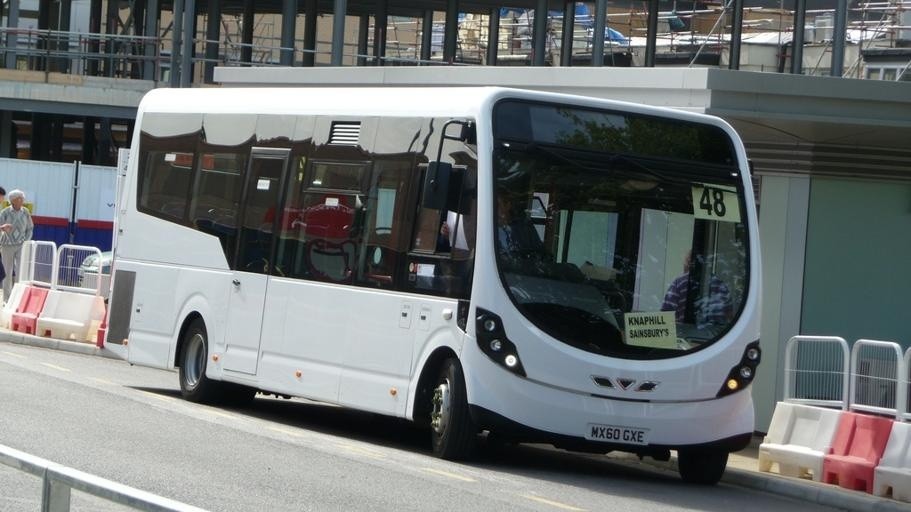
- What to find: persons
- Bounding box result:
[0,189,34,307]
[441,190,545,274]
[0,186,6,204]
[636,251,733,462]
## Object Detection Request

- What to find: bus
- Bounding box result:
[103,86,772,486]
